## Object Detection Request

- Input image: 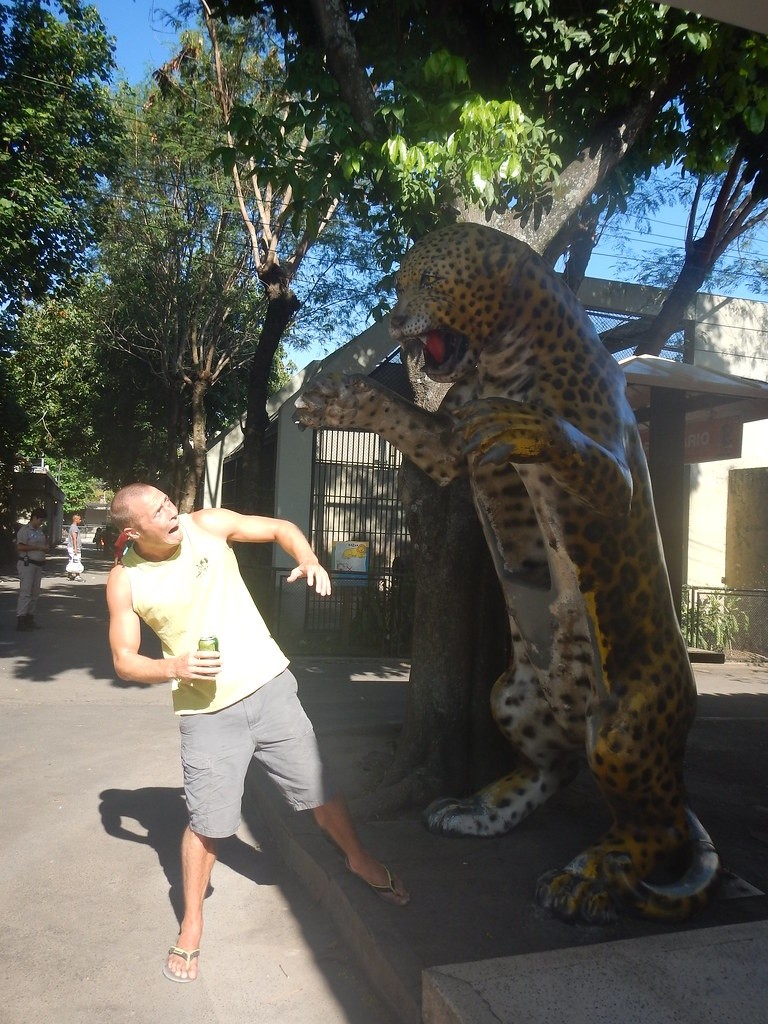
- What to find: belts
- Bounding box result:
[18,557,45,566]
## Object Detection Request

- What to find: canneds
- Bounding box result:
[198,636,220,676]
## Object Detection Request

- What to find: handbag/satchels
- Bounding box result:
[65,553,84,573]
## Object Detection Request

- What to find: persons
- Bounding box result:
[107,484,413,982]
[67,515,87,583]
[94,527,130,566]
[17,508,51,632]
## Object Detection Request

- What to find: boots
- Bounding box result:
[16,616,34,632]
[27,614,41,631]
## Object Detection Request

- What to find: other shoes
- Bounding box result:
[73,575,86,583]
[66,575,75,581]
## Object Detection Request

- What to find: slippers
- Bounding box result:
[345,855,410,906]
[163,946,200,983]
